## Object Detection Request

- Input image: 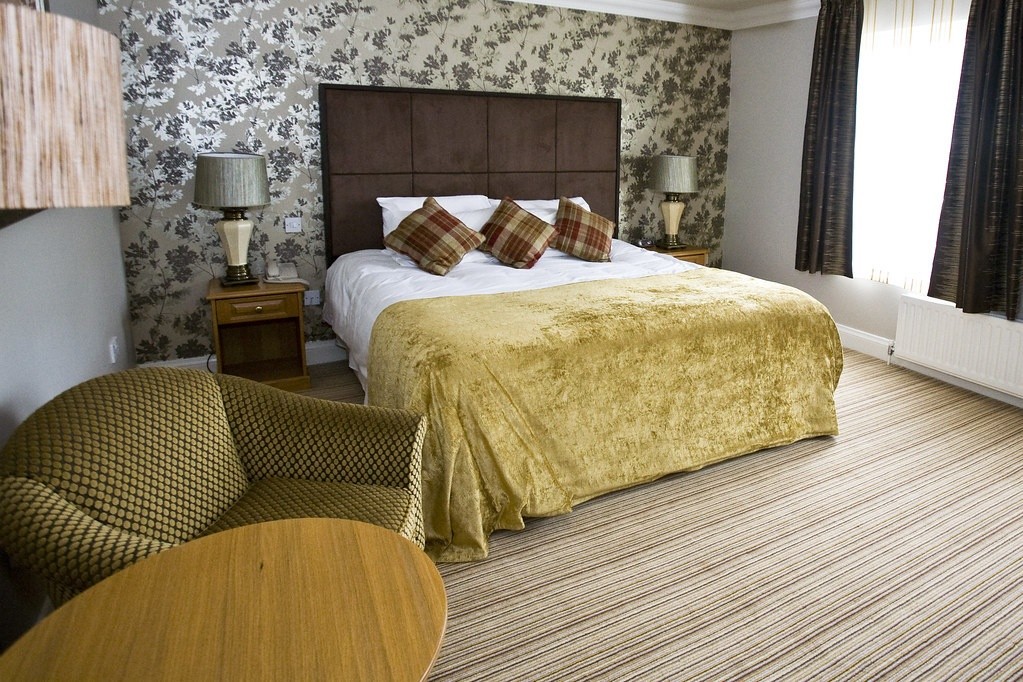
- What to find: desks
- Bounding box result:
[0,517,448,682]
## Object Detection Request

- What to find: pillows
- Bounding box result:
[475,196,561,269]
[548,195,616,262]
[382,197,487,276]
[376,195,491,248]
[385,204,497,268]
[488,196,591,250]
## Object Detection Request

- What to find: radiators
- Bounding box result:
[886,293,1023,400]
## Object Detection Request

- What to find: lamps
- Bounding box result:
[0,3,130,229]
[649,154,699,250]
[191,151,272,289]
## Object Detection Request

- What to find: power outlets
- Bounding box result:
[304,290,320,306]
[109,336,121,363]
[285,217,301,233]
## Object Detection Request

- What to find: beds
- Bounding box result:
[318,82,844,564]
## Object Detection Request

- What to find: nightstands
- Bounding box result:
[645,245,710,266]
[206,274,312,391]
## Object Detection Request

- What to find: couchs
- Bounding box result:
[0,366,430,608]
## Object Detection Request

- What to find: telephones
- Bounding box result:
[262,261,310,285]
